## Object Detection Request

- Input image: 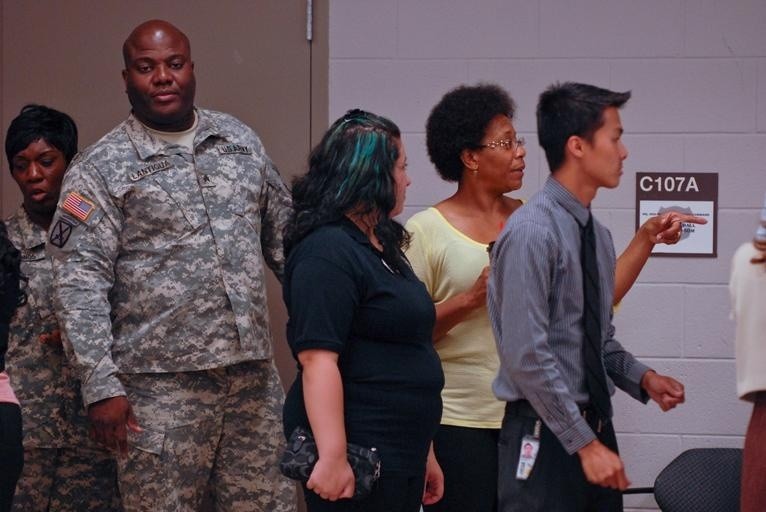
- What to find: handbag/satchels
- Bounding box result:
[279,426,381,500]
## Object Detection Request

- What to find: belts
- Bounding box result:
[505,399,593,421]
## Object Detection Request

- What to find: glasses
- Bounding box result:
[480,137,525,152]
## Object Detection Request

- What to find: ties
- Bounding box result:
[580,213,613,431]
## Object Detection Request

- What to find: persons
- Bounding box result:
[46,19,297,512]
[1,219,28,511]
[276,108,445,512]
[3,103,122,512]
[485,79,685,512]
[728,201,766,511]
[400,80,709,512]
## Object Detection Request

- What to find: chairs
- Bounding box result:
[652,446,745,511]
